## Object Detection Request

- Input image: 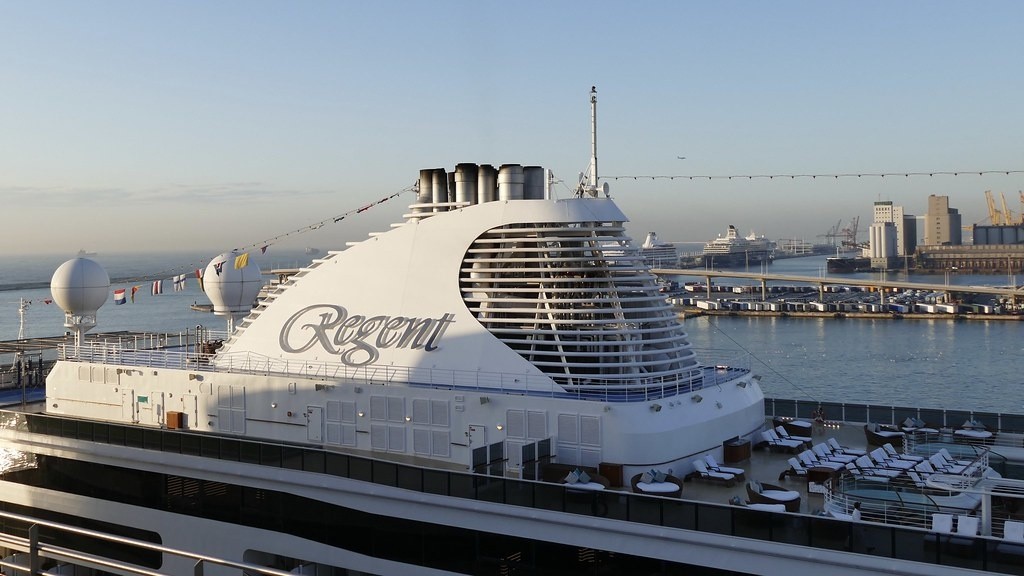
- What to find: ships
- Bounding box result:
[702,225,776,266]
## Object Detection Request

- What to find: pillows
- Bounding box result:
[653,469,668,483]
[874,420,880,432]
[974,420,987,433]
[572,468,582,478]
[638,472,655,484]
[578,471,591,484]
[649,468,657,477]
[564,471,578,485]
[914,417,927,428]
[755,480,763,494]
[971,420,977,426]
[961,419,973,432]
[750,479,759,493]
[902,417,914,428]
[911,418,916,423]
[867,420,875,433]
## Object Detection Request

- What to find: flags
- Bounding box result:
[131,286,141,304]
[214,261,226,276]
[235,253,249,270]
[151,280,164,296]
[173,274,186,292]
[196,268,205,292]
[114,289,126,305]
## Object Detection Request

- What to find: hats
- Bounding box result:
[854,501,861,507]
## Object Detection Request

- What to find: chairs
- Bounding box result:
[556,411,1024,568]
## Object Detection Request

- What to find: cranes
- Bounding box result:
[816,216,860,247]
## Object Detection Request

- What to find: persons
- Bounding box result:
[852,502,861,521]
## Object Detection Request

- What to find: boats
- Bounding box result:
[588,231,681,270]
[252,268,300,308]
[0,163,1024,575]
